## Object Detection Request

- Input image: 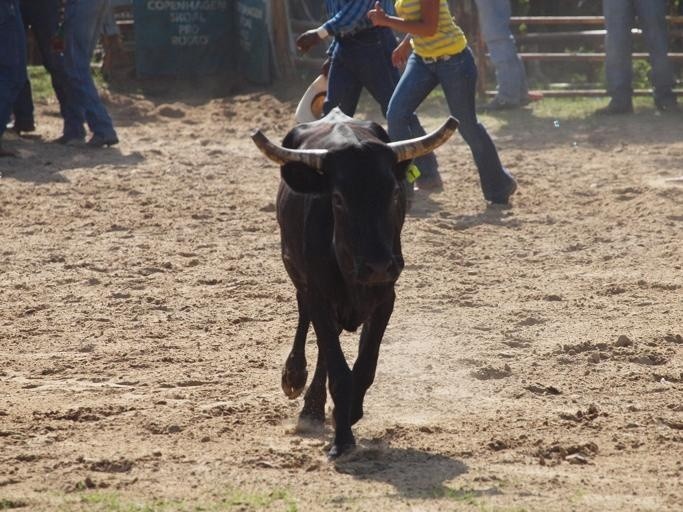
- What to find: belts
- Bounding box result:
[422,54,449,65]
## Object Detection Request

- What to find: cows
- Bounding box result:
[249,104,460,463]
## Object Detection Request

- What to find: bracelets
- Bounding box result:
[317,27,329,39]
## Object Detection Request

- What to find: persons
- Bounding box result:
[367,0,517,206]
[24,0,119,146]
[0,0,42,156]
[592,0,678,115]
[294,0,442,191]
[473,0,529,111]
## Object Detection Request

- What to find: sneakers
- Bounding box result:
[658,98,677,113]
[7,121,35,132]
[596,102,632,115]
[52,130,119,147]
[478,96,529,111]
